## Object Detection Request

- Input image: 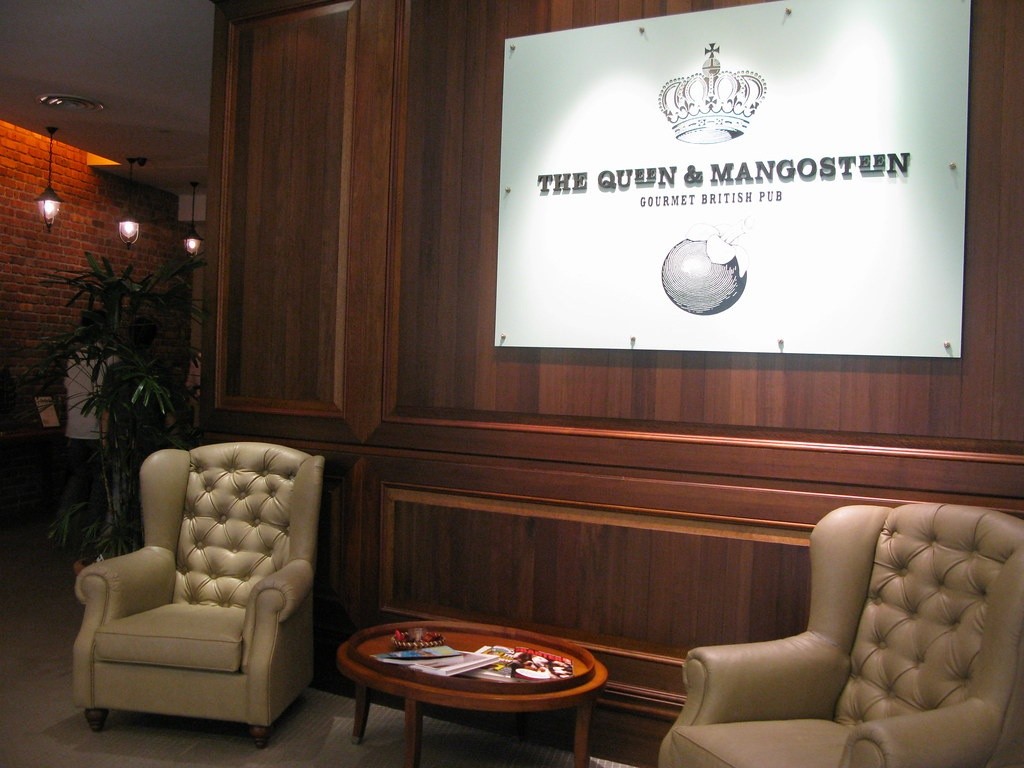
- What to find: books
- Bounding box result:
[371,643,526,683]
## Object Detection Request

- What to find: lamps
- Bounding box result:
[182,181,205,259]
[116,157,141,250]
[33,126,64,234]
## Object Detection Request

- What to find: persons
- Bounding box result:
[183,352,201,430]
[62,309,125,525]
[93,319,187,537]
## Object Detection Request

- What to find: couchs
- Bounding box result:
[656,504,1024,768]
[74,441,326,750]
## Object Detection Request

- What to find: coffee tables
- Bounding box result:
[336,620,609,767]
